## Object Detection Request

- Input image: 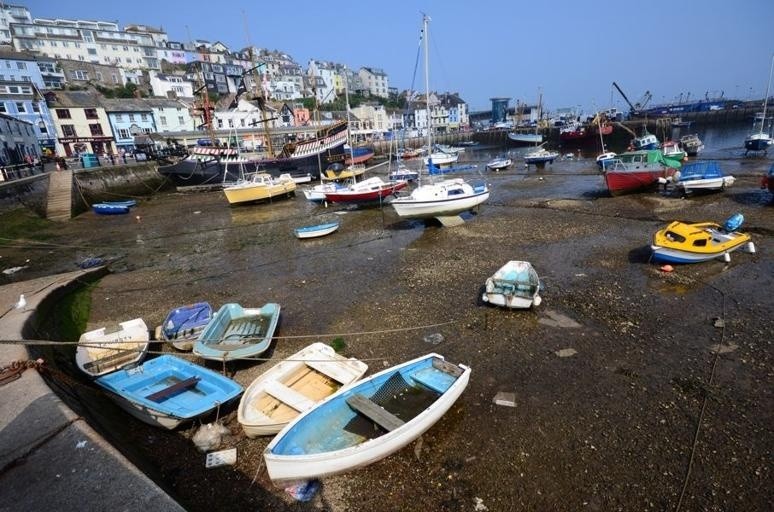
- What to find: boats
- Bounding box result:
[92,199,137,215]
[74,259,544,488]
